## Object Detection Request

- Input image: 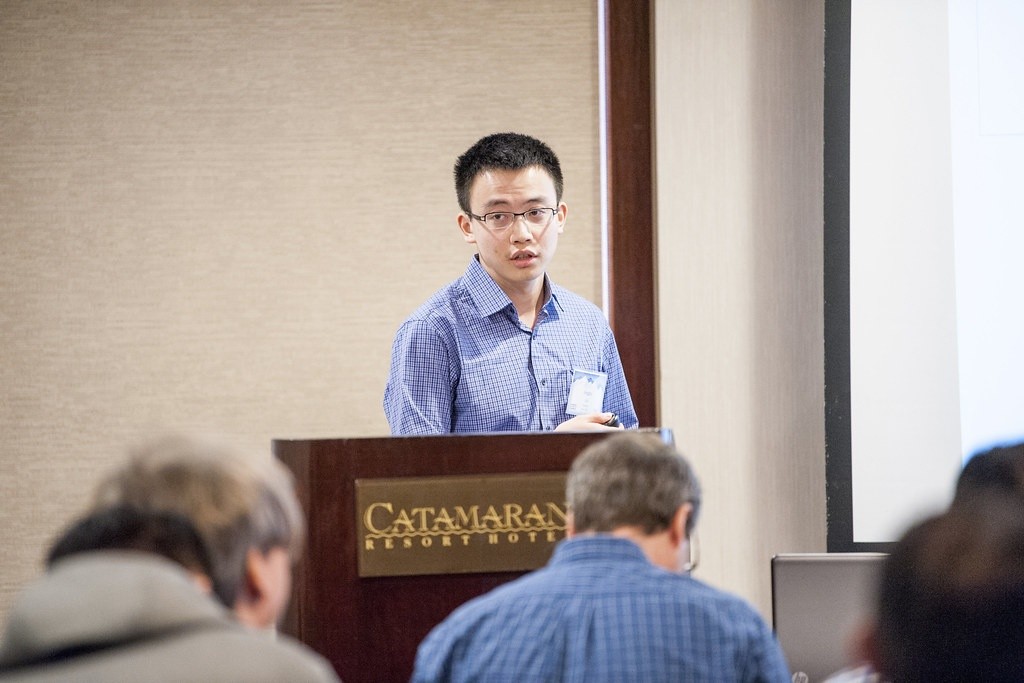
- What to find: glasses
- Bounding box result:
[468,207,559,229]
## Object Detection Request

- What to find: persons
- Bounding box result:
[819,443,1024,682]
[414,431,793,682]
[384,131,639,434]
[0,436,337,682]
